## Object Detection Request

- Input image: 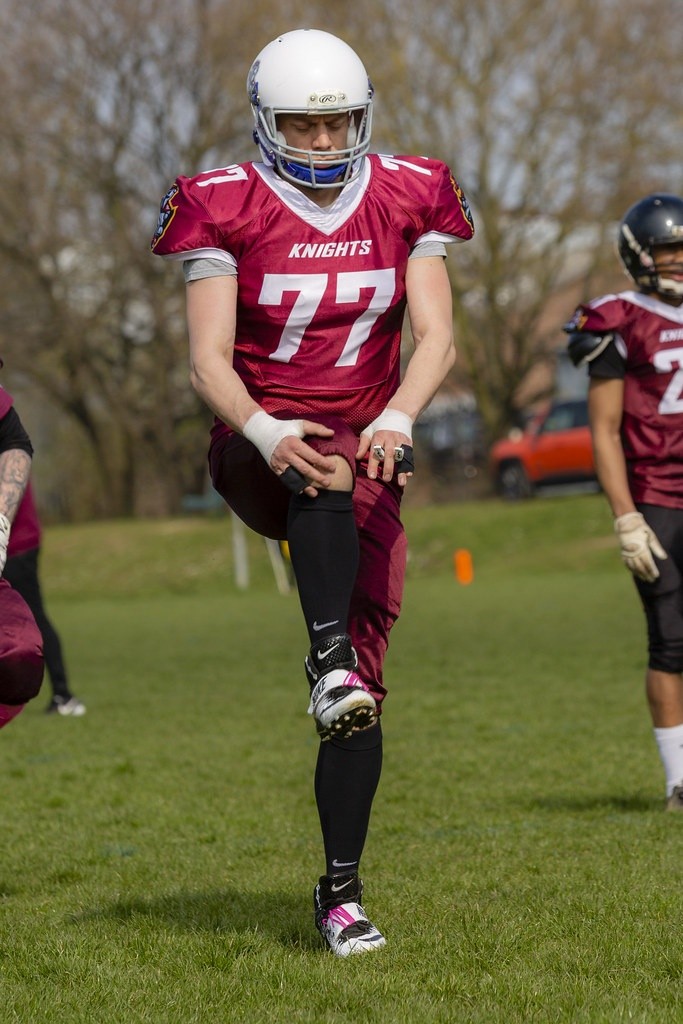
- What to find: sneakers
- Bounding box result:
[314,870,388,958]
[303,634,378,742]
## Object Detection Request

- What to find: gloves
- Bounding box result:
[0,514,11,578]
[614,512,670,584]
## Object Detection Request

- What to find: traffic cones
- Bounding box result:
[456,551,473,582]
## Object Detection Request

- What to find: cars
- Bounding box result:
[489,395,605,502]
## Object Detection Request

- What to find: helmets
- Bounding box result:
[246,28,374,170]
[620,195,683,293]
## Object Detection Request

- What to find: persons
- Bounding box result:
[149,29,476,953]
[422,420,476,477]
[561,195,682,814]
[0,353,84,736]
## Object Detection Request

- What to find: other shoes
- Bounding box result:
[45,695,88,716]
